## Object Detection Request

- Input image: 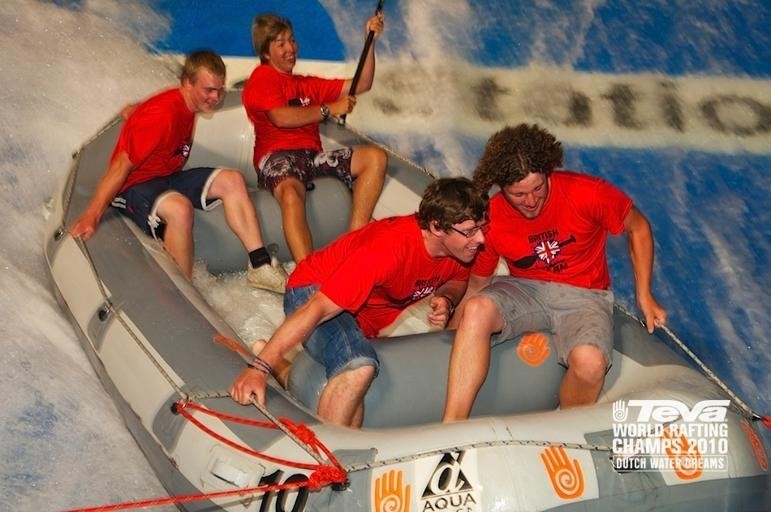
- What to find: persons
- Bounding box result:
[242,12,388,264]
[228,176,492,427]
[442,123,667,421]
[70,51,290,295]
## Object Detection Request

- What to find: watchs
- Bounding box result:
[320,102,330,120]
[444,296,454,316]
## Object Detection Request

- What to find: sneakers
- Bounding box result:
[247,256,288,293]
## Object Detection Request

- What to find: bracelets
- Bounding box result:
[248,356,272,374]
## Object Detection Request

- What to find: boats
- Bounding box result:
[42,89,771,512]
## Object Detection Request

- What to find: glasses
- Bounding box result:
[448,213,491,238]
